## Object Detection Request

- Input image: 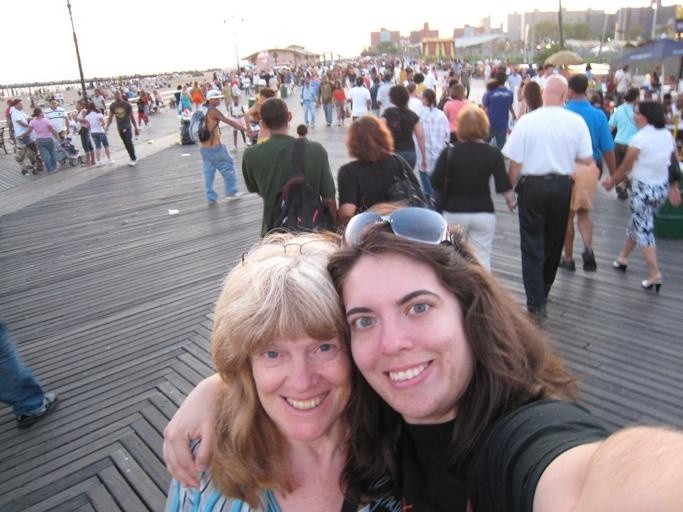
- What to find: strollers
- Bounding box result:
[56,134,82,167]
[10,136,44,174]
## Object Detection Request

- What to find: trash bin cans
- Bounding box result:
[281,86,288,98]
[653,166,683,240]
[249,98,256,108]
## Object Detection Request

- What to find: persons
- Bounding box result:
[500,75,594,323]
[0,318,57,430]
[162,199,683,512]
[242,99,339,236]
[428,103,516,275]
[165,229,374,512]
[602,102,681,292]
[335,117,424,228]
[558,76,616,272]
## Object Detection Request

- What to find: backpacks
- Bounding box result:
[187,104,211,142]
[269,172,334,234]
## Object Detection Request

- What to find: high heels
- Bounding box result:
[639,279,663,294]
[610,258,629,274]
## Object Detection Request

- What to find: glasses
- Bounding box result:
[343,206,454,249]
[239,238,341,268]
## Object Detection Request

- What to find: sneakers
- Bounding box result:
[14,392,58,431]
[556,254,576,272]
[580,246,597,272]
[127,160,137,167]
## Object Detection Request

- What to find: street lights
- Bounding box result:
[651,3,658,40]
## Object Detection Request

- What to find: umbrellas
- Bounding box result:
[542,50,588,72]
[628,37,683,102]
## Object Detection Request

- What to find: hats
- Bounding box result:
[206,89,224,101]
